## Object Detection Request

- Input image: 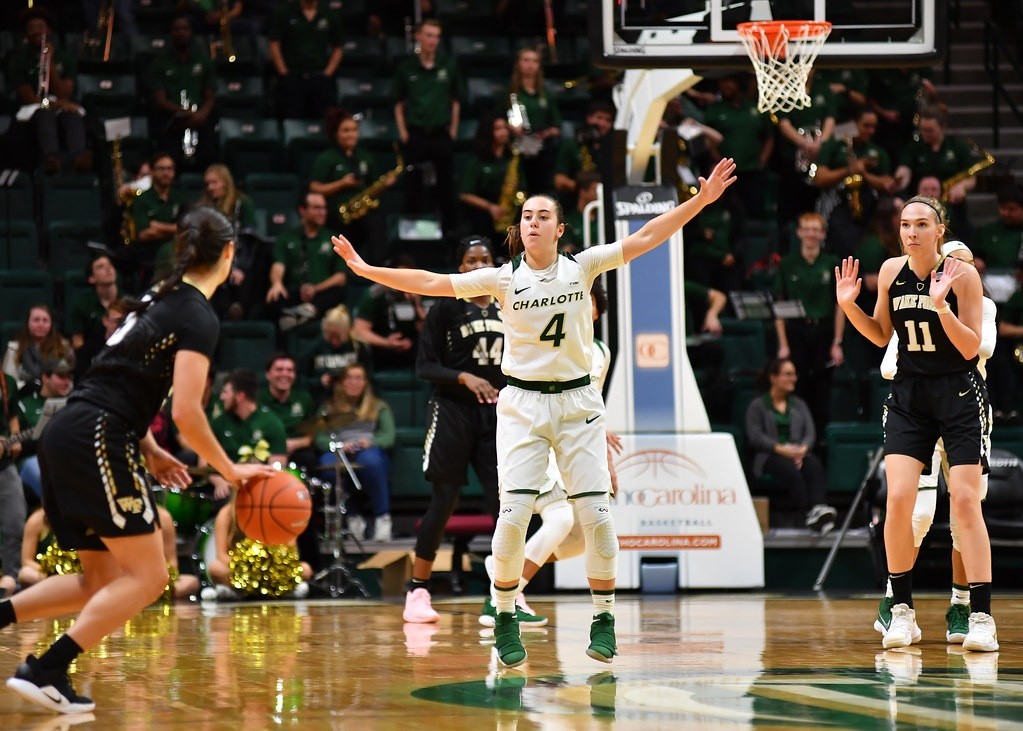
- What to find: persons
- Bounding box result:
[331,158,737,668]
[0,0,1023,643]
[834,194,1000,651]
[0,206,277,714]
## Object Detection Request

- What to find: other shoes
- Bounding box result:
[286,583,309,597]
[348,521,365,538]
[217,586,240,597]
[375,521,392,542]
[807,506,836,535]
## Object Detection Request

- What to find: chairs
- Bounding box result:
[0,0,1023,546]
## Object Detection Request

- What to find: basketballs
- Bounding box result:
[236,472,312,544]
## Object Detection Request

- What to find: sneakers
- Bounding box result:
[402,587,439,623]
[875,644,923,686]
[485,555,535,614]
[479,627,549,645]
[3,712,97,731]
[947,643,967,656]
[478,595,548,627]
[494,608,528,669]
[962,652,1000,687]
[874,594,922,643]
[403,622,439,656]
[586,612,619,663]
[493,669,527,718]
[962,612,1000,652]
[945,603,971,643]
[585,670,616,716]
[5,652,95,712]
[882,603,915,648]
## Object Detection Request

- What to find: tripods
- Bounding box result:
[309,442,372,600]
